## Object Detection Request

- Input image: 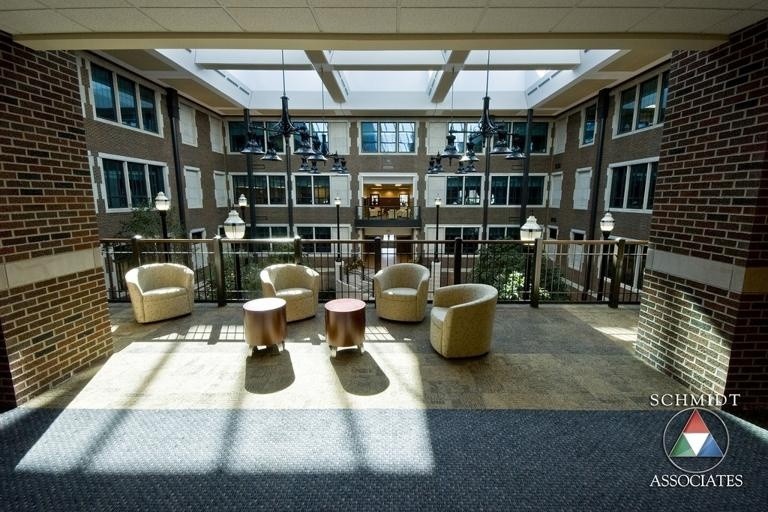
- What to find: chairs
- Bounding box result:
[124,262,195,323]
[429,282,499,360]
[368,206,411,220]
[373,262,430,325]
[259,262,321,323]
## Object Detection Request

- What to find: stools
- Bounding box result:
[243,297,288,357]
[324,297,367,359]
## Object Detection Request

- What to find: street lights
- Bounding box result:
[154,190,169,261]
[434,193,441,261]
[334,193,342,260]
[520,216,540,300]
[597,211,614,301]
[238,193,247,221]
[224,208,244,298]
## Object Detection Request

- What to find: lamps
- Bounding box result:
[240,49,528,174]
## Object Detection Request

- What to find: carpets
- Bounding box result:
[0,406,767,512]
